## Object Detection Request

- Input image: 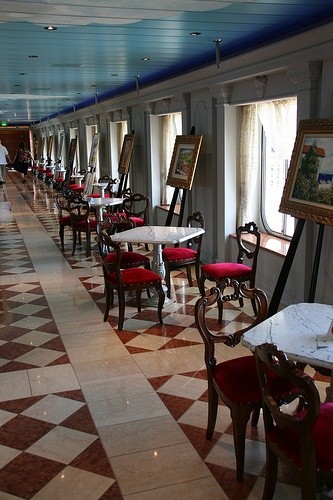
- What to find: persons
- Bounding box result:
[0,139,12,185]
[12,142,36,182]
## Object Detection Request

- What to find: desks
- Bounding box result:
[54,170,67,180]
[80,198,123,235]
[241,303,333,370]
[46,166,56,173]
[70,175,85,187]
[89,183,110,198]
[109,226,205,309]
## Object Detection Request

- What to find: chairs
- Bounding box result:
[30,159,333,500]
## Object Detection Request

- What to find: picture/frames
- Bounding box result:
[47,135,54,159]
[88,133,101,168]
[278,118,333,226]
[67,139,77,164]
[165,135,203,191]
[118,133,135,174]
